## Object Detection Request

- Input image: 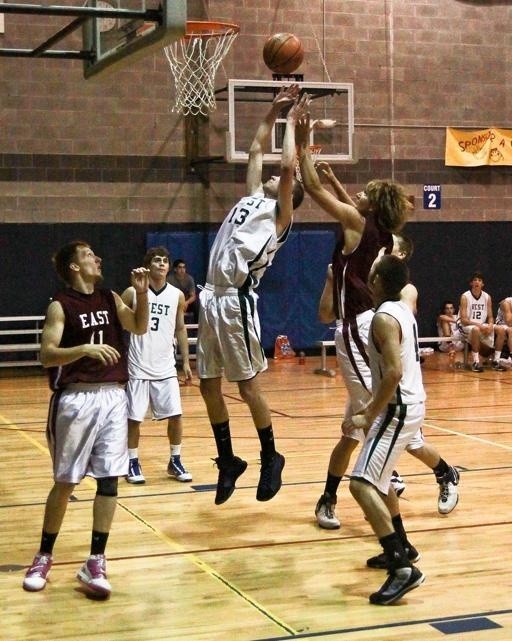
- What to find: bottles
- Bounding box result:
[299,351,305,365]
[448,349,457,368]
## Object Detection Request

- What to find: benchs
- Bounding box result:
[314,334,476,378]
[0,313,201,369]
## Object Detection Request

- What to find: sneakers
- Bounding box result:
[472,361,483,372]
[448,349,457,358]
[436,466,460,514]
[256,450,285,501]
[390,470,406,497]
[315,494,341,530]
[366,544,420,568]
[369,564,425,605]
[23,550,53,592]
[126,457,145,484]
[491,361,506,371]
[76,553,112,596]
[213,456,248,505]
[166,453,192,482]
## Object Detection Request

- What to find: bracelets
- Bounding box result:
[136,290,148,295]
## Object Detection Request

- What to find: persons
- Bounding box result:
[196,83,313,504]
[22,240,151,597]
[167,259,196,324]
[494,297,512,358]
[316,231,418,529]
[340,253,421,606]
[456,272,507,372]
[294,109,460,515]
[119,246,192,484]
[437,302,465,357]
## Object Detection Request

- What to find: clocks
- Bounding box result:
[83,0,118,33]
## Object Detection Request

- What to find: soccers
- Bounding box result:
[264,31,304,74]
